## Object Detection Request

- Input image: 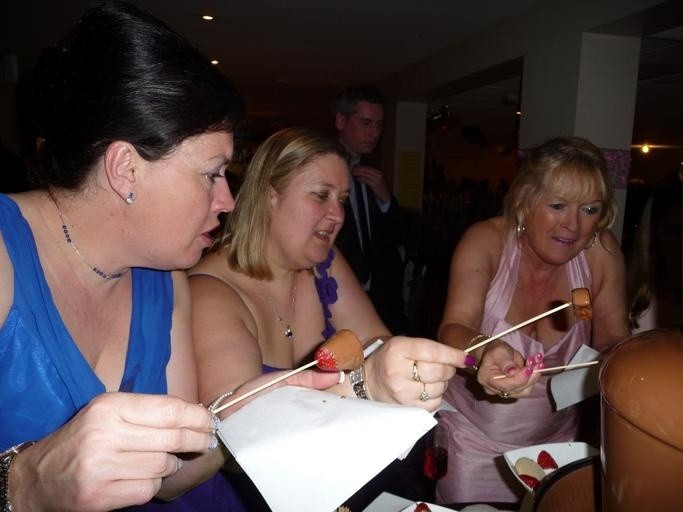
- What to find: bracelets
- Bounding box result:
[0,436,39,512]
[348,364,367,400]
[467,332,487,372]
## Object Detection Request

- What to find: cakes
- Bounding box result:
[572,288,593,320]
[536,329,683,512]
[314,329,364,372]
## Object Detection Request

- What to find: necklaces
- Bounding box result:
[47,188,133,280]
[251,262,300,341]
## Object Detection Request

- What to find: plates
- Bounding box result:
[399,501,460,512]
[502,441,599,503]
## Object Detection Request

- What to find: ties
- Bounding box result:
[351,164,371,286]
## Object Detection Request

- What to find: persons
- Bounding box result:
[432,133,633,512]
[0,1,238,512]
[318,83,413,319]
[622,171,682,341]
[184,124,479,511]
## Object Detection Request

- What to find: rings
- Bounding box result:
[412,359,419,382]
[499,389,510,399]
[419,382,428,402]
